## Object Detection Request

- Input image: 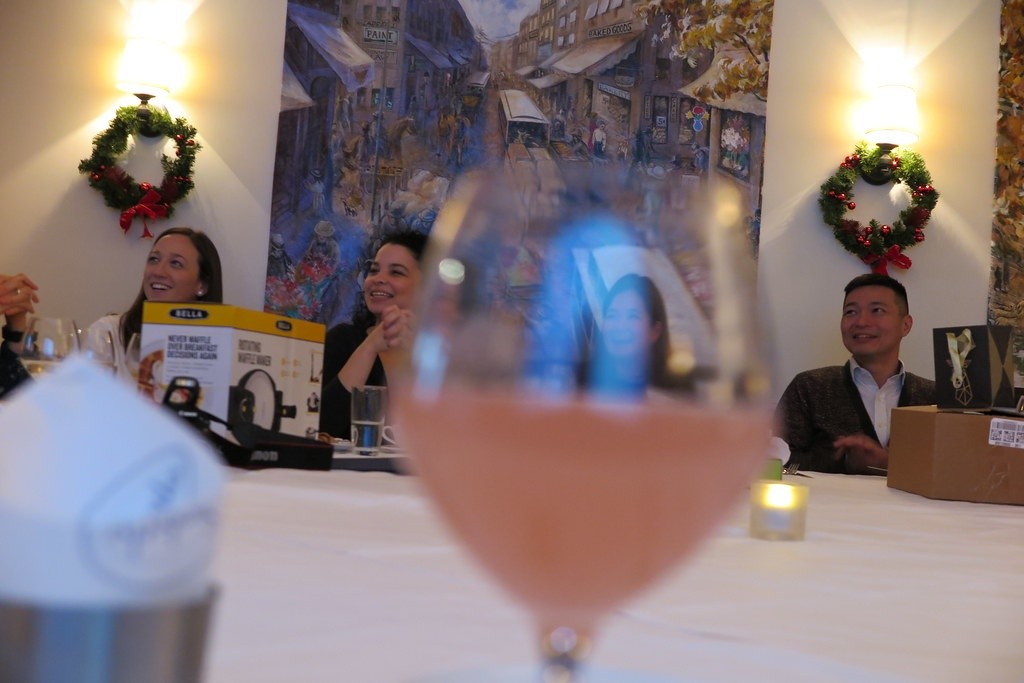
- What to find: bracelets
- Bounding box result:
[2,325,39,343]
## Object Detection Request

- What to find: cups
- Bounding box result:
[123,334,143,384]
[349,384,387,449]
[18,314,82,389]
[76,325,117,380]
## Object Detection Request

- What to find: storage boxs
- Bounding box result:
[140,297,325,448]
[885,405,1024,505]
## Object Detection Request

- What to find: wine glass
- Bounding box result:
[381,152,785,683]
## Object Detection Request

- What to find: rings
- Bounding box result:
[17,289,20,295]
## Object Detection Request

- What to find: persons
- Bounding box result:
[770,274,936,476]
[319,229,457,445]
[559,274,695,399]
[0,273,40,402]
[83,226,223,387]
